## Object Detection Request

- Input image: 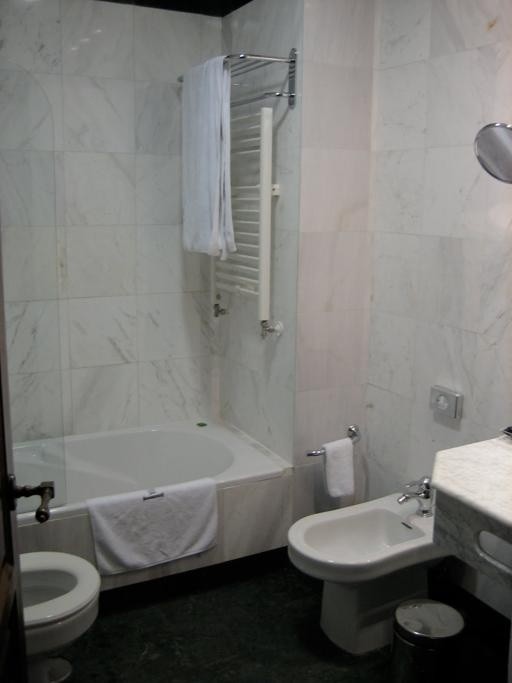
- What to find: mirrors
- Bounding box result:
[475,122,512,184]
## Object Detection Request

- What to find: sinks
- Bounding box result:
[286,488,453,583]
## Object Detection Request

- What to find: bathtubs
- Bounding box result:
[12,425,295,592]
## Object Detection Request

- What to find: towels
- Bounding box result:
[88,476,221,580]
[177,56,237,257]
[323,436,355,500]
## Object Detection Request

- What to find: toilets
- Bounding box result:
[19,550,102,683]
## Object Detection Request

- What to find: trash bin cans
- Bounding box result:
[27,657,73,683]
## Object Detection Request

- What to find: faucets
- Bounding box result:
[398,478,434,518]
